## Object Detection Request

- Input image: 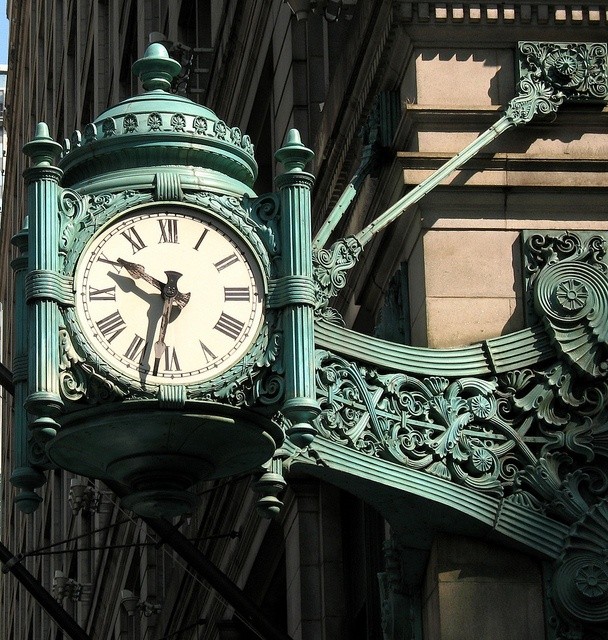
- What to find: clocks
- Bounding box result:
[74,202,269,388]
